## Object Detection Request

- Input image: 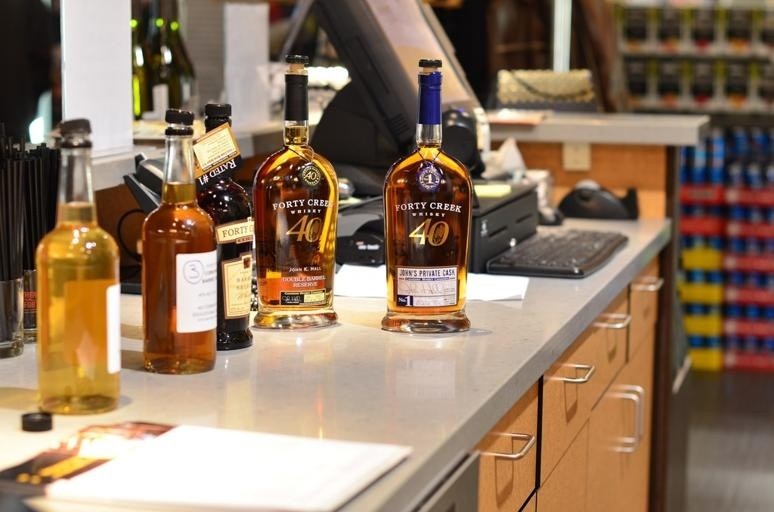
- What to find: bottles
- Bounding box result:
[251,54,340,334]
[33,113,122,417]
[614,1,773,112]
[139,107,221,376]
[380,57,472,334]
[131,1,199,125]
[190,102,256,352]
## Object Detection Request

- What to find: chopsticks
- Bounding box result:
[0,120,62,343]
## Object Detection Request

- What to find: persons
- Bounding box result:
[0,0,63,144]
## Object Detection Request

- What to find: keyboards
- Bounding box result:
[487,228,629,279]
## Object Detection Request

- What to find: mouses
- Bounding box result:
[539,205,565,225]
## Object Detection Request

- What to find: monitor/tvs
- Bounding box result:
[308,0,482,152]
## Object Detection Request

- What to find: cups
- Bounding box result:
[0,275,24,358]
[19,269,38,340]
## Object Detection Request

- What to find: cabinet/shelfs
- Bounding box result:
[615,2,773,370]
[475,244,662,512]
